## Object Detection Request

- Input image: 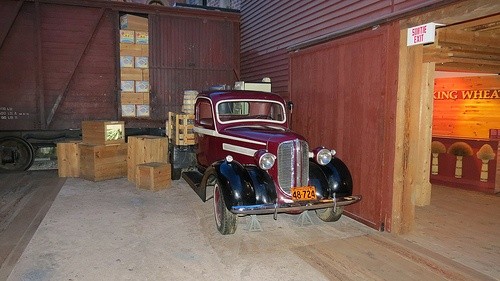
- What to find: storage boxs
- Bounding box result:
[120,67,142,81]
[79,143,127,182]
[127,135,168,185]
[143,92,149,104]
[82,119,126,146]
[121,93,143,105]
[120,55,134,68]
[120,30,134,44]
[135,31,149,45]
[56,141,79,178]
[143,69,149,81]
[122,104,136,117]
[120,14,148,32]
[135,56,149,69]
[121,80,136,93]
[135,80,149,93]
[120,43,149,57]
[136,104,149,117]
[136,161,171,193]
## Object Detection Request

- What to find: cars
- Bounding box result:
[171,88,361,235]
